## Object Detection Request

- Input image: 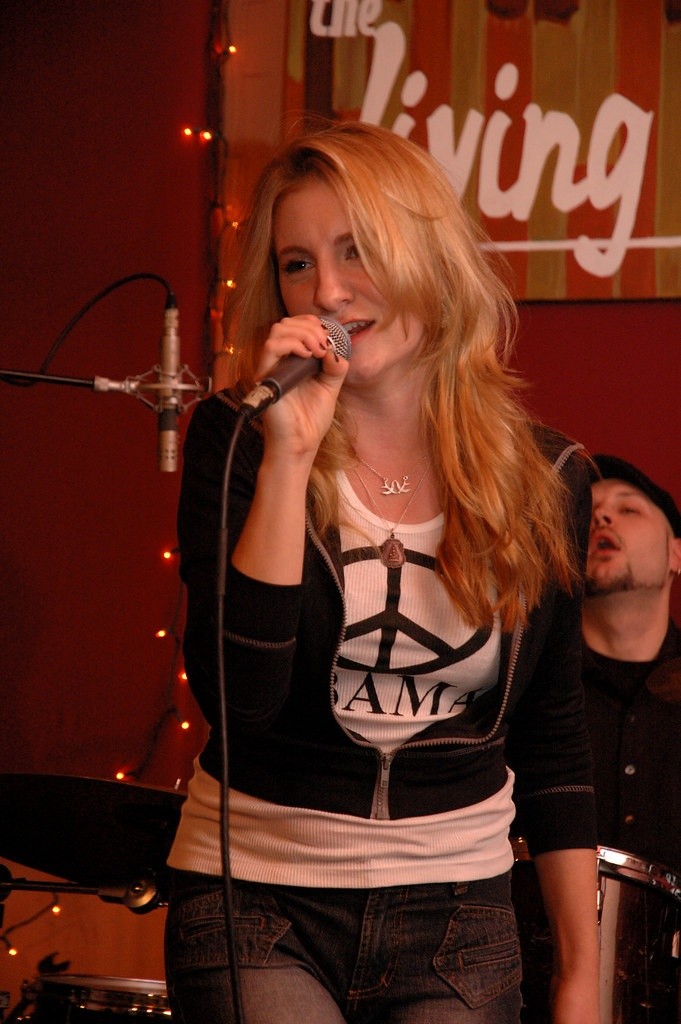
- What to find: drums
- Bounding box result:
[32,975,172,1024]
[510,835,681,1024]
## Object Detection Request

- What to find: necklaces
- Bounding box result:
[347,434,437,569]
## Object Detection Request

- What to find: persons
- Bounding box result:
[498,451,681,1024]
[157,116,604,1024]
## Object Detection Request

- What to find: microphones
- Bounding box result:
[157,296,179,474]
[239,317,353,415]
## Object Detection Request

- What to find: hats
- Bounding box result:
[587,453,681,538]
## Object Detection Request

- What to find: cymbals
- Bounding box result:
[1,769,188,890]
